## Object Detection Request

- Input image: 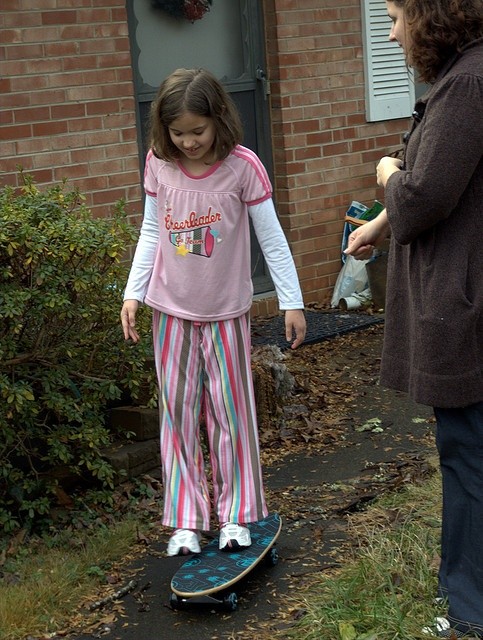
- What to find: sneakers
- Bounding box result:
[221,521,252,551]
[421,616,483,640]
[167,528,201,559]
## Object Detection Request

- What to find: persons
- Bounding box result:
[121,68,308,558]
[343,0,482,640]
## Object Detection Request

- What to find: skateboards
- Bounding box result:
[170,514,282,611]
